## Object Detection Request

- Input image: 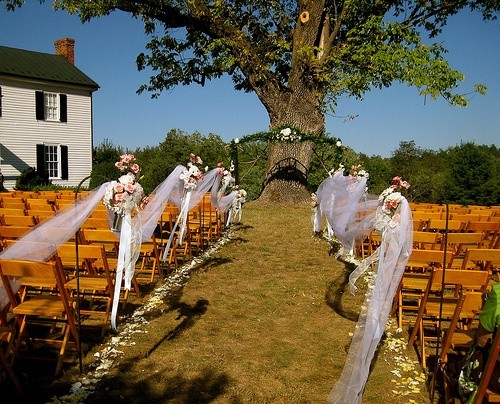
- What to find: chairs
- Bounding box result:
[0,188,230,383]
[351,193,500,404]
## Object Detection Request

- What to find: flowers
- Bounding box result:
[179,155,209,191]
[376,177,410,219]
[101,154,149,232]
[216,161,247,214]
[348,164,369,183]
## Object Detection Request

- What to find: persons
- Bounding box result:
[444,282,500,394]
